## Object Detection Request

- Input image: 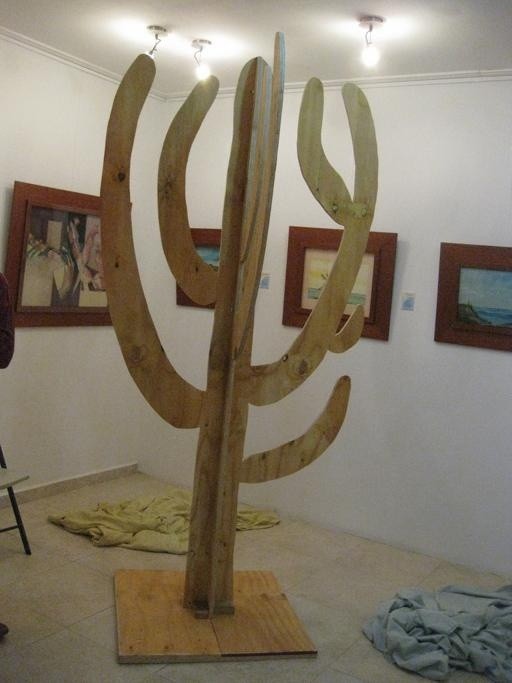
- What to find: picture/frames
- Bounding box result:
[434,241,512,351]
[3,180,114,327]
[282,226,398,342]
[176,228,221,308]
